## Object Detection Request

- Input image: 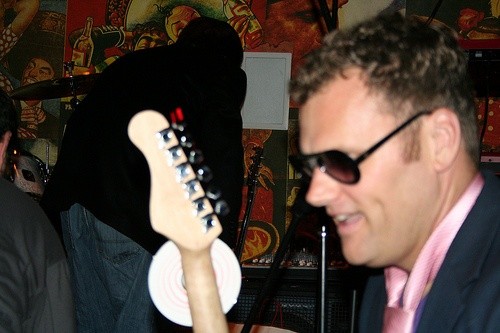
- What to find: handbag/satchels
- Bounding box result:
[6,14,246,254]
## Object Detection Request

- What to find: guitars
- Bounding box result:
[234,145,264,263]
[126,109,229,333]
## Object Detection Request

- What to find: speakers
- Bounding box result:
[227,266,360,333]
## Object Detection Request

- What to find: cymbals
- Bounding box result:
[9,73,100,100]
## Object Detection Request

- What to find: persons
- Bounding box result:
[288,13,499,333]
[0,87,78,333]
[43,17,248,333]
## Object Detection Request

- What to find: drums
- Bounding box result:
[7,149,50,203]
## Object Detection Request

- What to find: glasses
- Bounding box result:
[287,111,432,185]
